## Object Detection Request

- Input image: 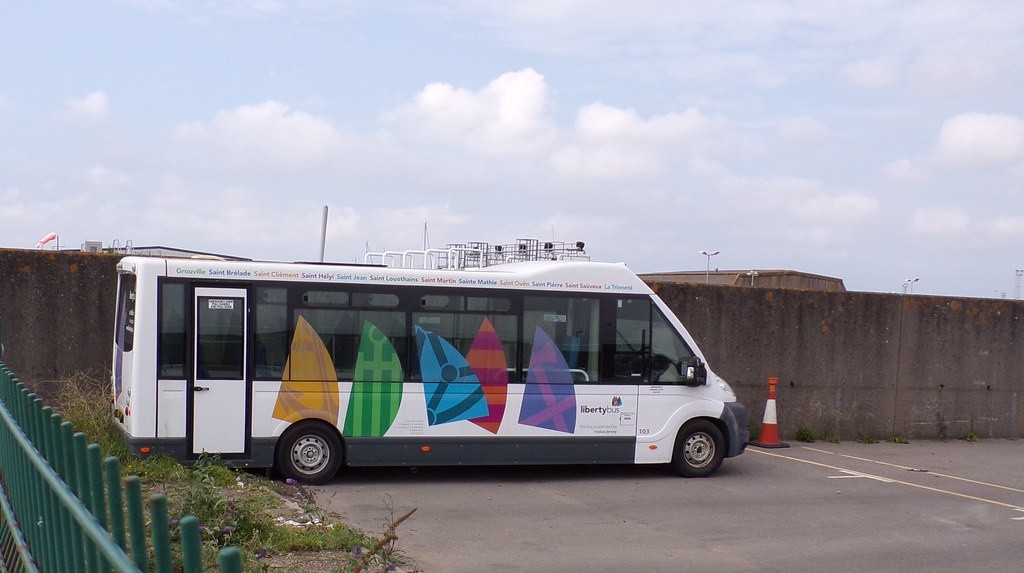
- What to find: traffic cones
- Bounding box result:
[747,378,791,448]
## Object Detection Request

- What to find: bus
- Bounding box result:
[106,255,747,486]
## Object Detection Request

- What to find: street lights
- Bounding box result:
[1014,269,1024,299]
[700,251,721,284]
[906,277,920,294]
[746,271,759,287]
